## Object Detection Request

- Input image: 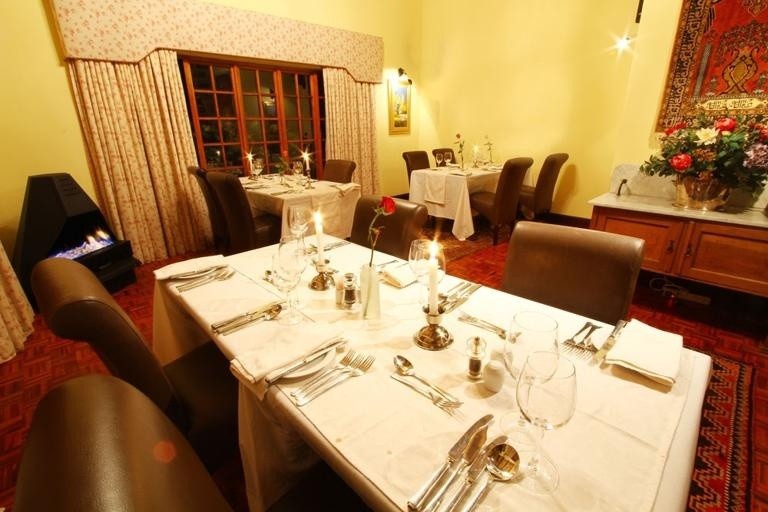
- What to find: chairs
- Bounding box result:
[403,150,429,195]
[206,171,281,252]
[320,159,356,183]
[351,197,427,261]
[519,154,568,221]
[501,221,645,326]
[433,148,455,170]
[187,166,229,255]
[30,256,238,437]
[471,157,534,246]
[14,375,368,511]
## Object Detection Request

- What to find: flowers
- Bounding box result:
[364,196,396,318]
[640,106,768,196]
[453,134,465,167]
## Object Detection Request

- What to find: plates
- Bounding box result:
[239,328,336,381]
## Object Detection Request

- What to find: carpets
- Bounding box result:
[687,343,755,512]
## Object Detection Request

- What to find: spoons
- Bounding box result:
[212,304,282,335]
[461,444,520,512]
[393,355,460,404]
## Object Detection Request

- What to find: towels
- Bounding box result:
[424,169,448,205]
[229,319,347,401]
[379,261,417,289]
[606,319,685,387]
[333,183,359,198]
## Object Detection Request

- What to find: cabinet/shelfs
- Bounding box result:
[589,193,768,299]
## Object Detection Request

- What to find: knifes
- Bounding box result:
[407,414,507,512]
[264,339,347,382]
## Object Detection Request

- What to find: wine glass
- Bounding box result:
[270,253,302,325]
[501,312,561,440]
[436,154,443,170]
[517,349,576,497]
[444,152,452,169]
[277,235,306,309]
[286,206,310,268]
[252,158,302,185]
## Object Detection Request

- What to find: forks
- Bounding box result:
[176,270,235,293]
[290,350,375,405]
[391,374,464,408]
[562,321,600,363]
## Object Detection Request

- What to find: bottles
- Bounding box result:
[466,335,512,393]
[334,272,360,308]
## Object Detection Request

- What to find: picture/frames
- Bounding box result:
[388,79,410,135]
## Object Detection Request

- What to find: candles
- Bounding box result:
[426,239,438,314]
[248,151,254,172]
[304,153,311,170]
[315,212,325,266]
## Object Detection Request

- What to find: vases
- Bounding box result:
[458,155,464,170]
[672,169,730,212]
[360,266,380,322]
